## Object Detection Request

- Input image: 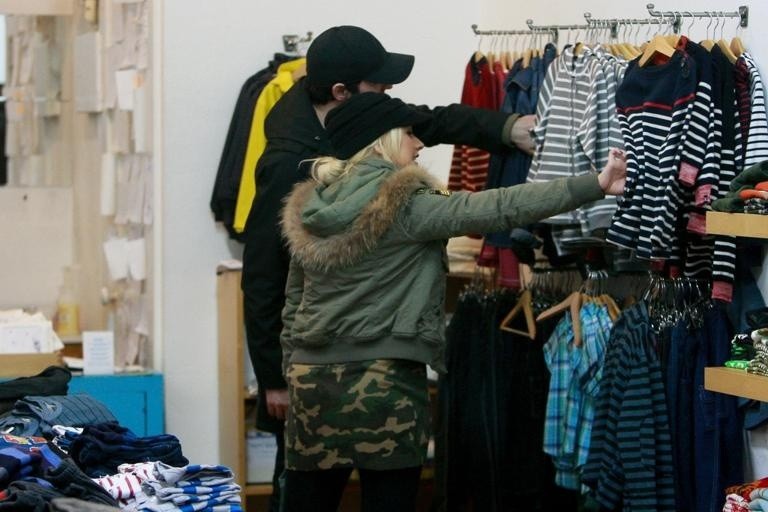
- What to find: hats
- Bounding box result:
[305,25,416,86]
[325,92,435,160]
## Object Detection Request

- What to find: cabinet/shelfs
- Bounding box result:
[703,211,768,404]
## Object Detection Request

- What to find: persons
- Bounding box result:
[274,89,632,510]
[241,22,553,511]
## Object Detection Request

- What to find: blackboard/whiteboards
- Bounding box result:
[2,186,75,310]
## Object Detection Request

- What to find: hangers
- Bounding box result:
[457,264,714,346]
[475,10,746,75]
